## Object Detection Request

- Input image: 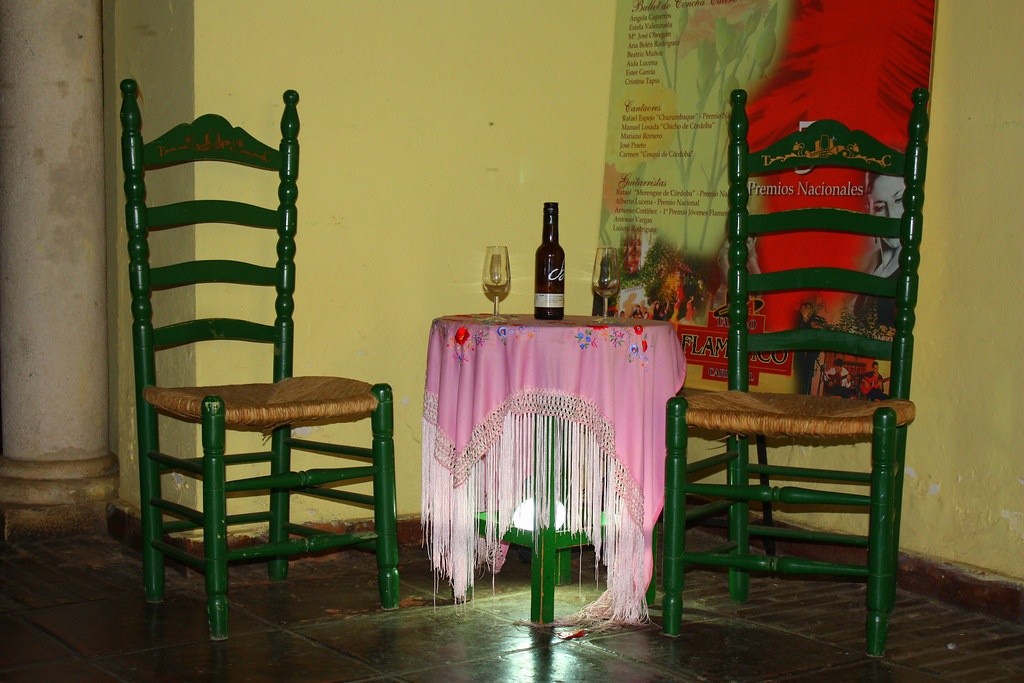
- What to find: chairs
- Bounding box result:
[658,88,929,659]
[119,77,403,641]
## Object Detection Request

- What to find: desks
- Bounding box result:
[419,313,689,631]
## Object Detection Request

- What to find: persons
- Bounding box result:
[794,174,907,401]
[612,295,699,322]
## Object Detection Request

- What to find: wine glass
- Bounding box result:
[591,247,622,323]
[482,245,511,323]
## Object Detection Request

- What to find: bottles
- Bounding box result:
[534,202,565,319]
